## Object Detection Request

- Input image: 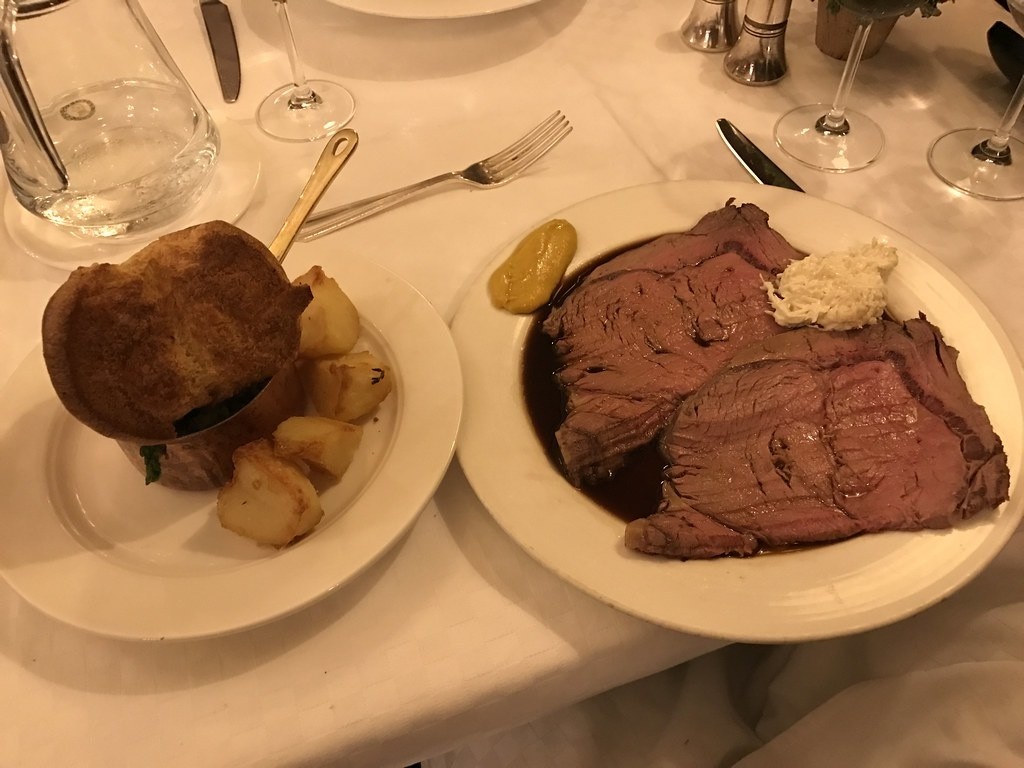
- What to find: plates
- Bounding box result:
[326,1,543,20]
[450,180,1024,648]
[0,241,467,646]
[3,112,267,269]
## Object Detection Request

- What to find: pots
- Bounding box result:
[113,128,359,492]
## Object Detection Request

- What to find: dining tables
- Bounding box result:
[0,1,1024,767]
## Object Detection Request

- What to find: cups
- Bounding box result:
[0,0,220,237]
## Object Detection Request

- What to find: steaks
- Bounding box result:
[612,316,1013,557]
[551,193,807,473]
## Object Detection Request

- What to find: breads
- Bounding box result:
[37,213,314,446]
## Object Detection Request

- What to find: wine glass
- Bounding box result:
[253,1,353,140]
[770,0,933,175]
[926,0,1024,202]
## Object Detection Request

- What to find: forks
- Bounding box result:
[294,108,573,241]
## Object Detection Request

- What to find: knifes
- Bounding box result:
[199,0,242,105]
[715,117,804,193]
